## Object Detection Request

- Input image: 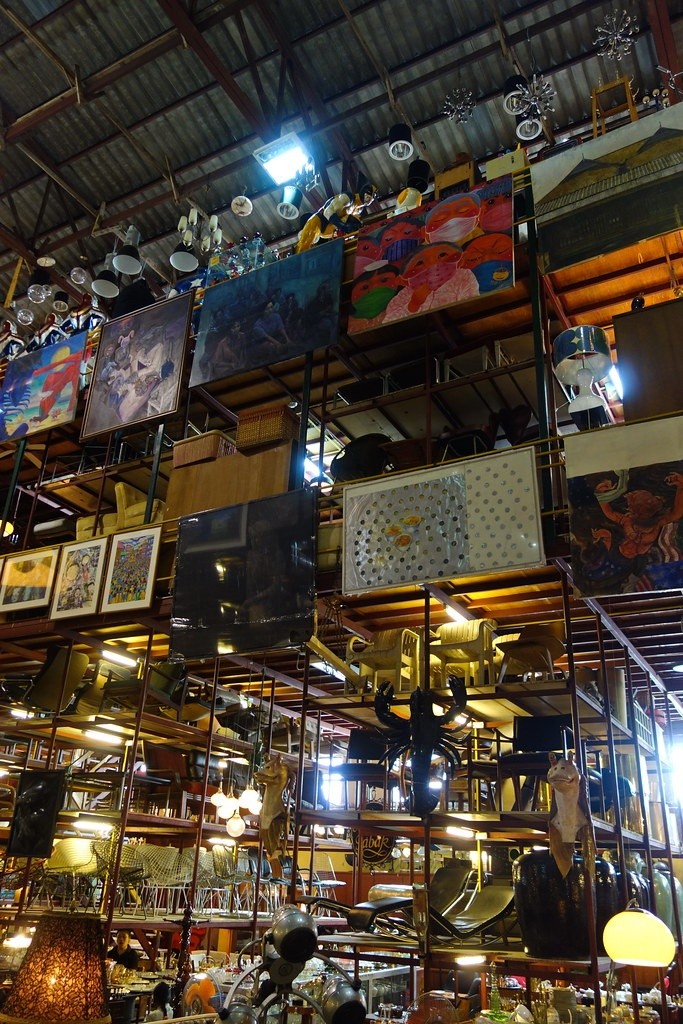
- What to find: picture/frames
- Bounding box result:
[0,545,62,613]
[99,524,164,614]
[48,534,111,622]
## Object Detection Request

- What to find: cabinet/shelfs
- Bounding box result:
[290,552,683,1024]
[0,618,350,1024]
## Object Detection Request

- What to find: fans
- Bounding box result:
[182,971,224,1016]
[404,992,460,1024]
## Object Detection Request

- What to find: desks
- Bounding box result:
[434,160,482,200]
[77,513,117,540]
[33,518,76,546]
[592,75,639,138]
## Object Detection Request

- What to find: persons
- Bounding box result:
[26,312,69,353]
[110,553,146,604]
[58,293,109,339]
[146,981,174,1022]
[0,320,26,365]
[241,518,280,631]
[57,582,94,611]
[107,928,139,1024]
[296,184,377,252]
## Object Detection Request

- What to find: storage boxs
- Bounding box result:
[486,148,532,181]
[172,430,237,468]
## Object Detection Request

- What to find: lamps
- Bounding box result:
[276,186,303,220]
[515,111,543,140]
[442,602,468,622]
[99,649,138,667]
[553,325,614,429]
[81,729,123,745]
[5,912,113,1024]
[643,88,670,113]
[209,653,264,837]
[444,827,475,838]
[178,184,223,255]
[388,124,414,161]
[252,131,315,185]
[53,291,69,311]
[602,898,676,1024]
[503,75,531,116]
[27,269,50,304]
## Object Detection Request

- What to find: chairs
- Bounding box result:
[0,838,516,950]
[0,648,332,836]
[328,713,635,822]
[343,625,425,694]
[430,618,566,687]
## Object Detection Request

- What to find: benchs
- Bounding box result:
[115,482,165,529]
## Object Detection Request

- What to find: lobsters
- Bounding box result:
[373,673,475,820]
